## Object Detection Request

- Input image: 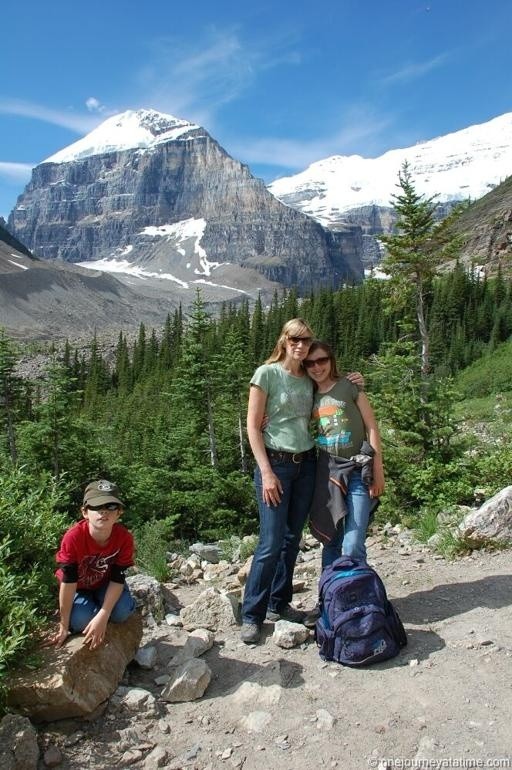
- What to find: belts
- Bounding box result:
[265,446,312,464]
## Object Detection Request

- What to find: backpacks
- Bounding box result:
[313,555,407,669]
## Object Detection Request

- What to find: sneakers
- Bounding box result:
[240,617,261,644]
[264,603,304,623]
[303,601,323,627]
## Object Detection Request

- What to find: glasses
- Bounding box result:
[285,330,313,347]
[303,356,330,368]
[86,503,119,512]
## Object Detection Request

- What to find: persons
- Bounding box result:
[258,340,389,628]
[238,316,365,645]
[39,479,138,652]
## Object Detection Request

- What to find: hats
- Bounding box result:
[82,479,126,508]
